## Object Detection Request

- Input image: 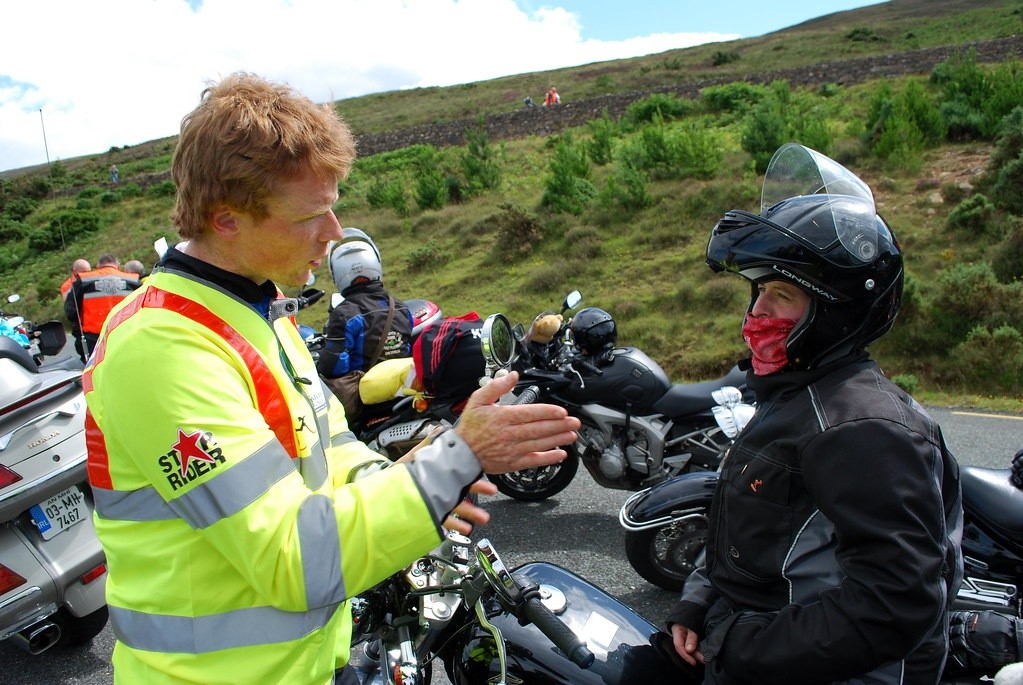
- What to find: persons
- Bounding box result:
[666,142,964,685]
[524,85,561,108]
[111,162,118,183]
[60,253,150,365]
[82,71,580,685]
[316,228,414,438]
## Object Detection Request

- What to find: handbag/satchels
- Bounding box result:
[412,312,486,402]
[316,370,364,417]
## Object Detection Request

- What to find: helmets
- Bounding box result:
[327,227,382,294]
[705,142,904,372]
[571,308,618,357]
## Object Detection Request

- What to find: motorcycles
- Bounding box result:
[1,294,109,654]
[269,271,1022,616]
[335,384,674,684]
